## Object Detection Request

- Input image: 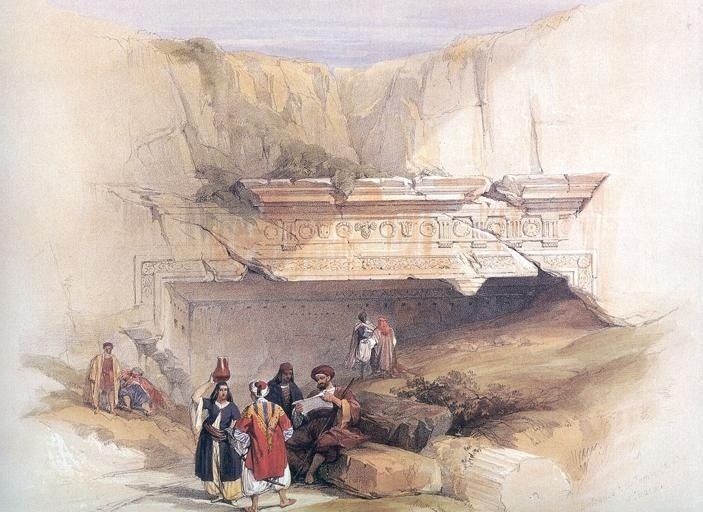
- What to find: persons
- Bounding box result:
[263,362,304,420]
[233,378,297,512]
[284,365,367,484]
[371,316,398,381]
[118,364,168,418]
[186,372,243,508]
[341,310,376,383]
[88,340,124,415]
